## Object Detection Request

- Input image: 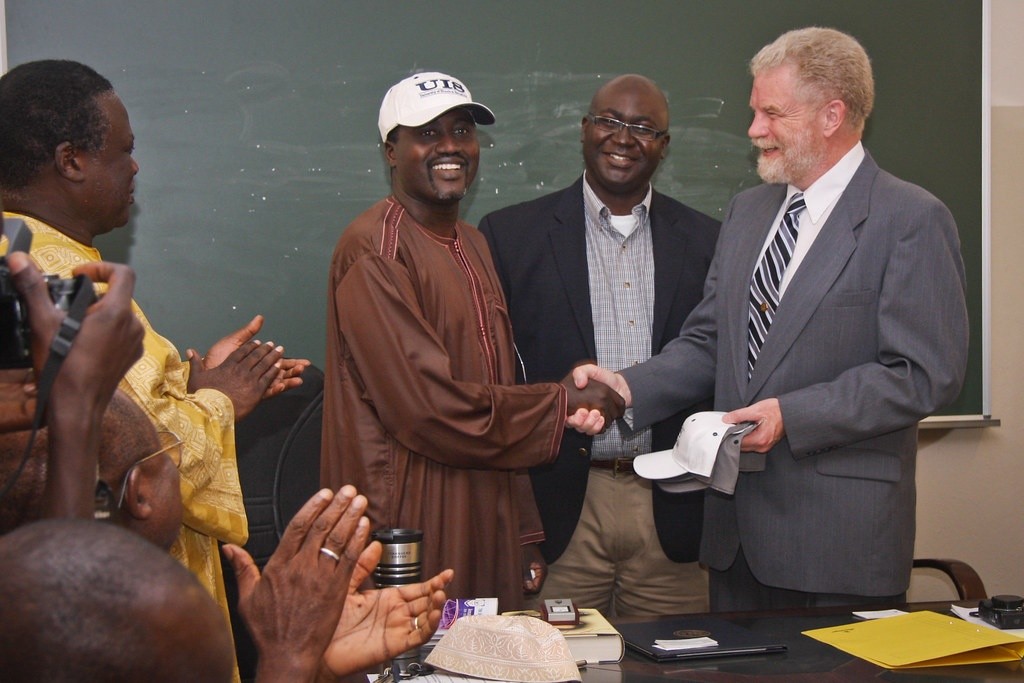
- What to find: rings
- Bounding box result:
[415,618,418,628]
[321,548,340,561]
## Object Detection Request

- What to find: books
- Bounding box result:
[504,609,625,663]
[613,615,788,662]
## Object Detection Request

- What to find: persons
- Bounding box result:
[1,59,310,683]
[1,517,235,683]
[477,75,720,616]
[0,383,455,682]
[568,29,969,611]
[318,72,628,615]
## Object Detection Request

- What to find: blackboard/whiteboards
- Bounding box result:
[5,1,991,429]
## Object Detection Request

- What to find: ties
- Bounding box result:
[747,193,806,383]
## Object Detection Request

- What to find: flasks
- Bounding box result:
[371,529,424,659]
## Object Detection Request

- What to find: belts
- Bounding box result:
[590,457,634,476]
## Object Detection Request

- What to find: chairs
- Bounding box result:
[225,358,323,622]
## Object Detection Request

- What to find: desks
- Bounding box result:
[337,596,1024,683]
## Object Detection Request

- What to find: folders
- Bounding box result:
[800,611,1024,670]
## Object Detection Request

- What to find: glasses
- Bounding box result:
[119,431,185,509]
[589,112,668,141]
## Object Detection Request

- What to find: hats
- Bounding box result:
[633,411,767,495]
[424,615,582,683]
[378,72,496,144]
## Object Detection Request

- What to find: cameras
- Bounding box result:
[0,216,82,370]
[979,594,1024,629]
